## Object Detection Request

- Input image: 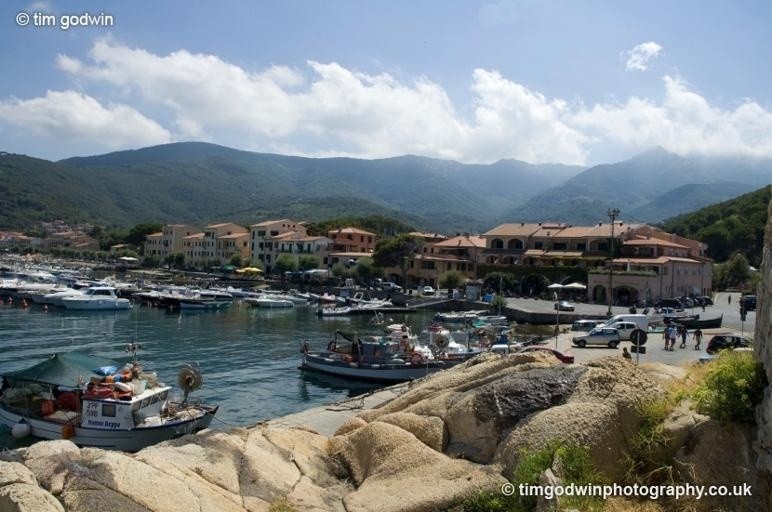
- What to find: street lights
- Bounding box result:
[606,206,619,315]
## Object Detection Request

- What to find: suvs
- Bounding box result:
[705,334,757,356]
[740,295,757,310]
[379,282,404,292]
[570,326,622,349]
[595,321,640,341]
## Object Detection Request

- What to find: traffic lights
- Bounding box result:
[744,300,748,315]
[739,300,743,314]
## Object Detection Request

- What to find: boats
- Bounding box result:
[1,349,218,453]
[432,310,482,323]
[297,327,496,382]
[415,323,539,356]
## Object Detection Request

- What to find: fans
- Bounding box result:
[177,368,203,408]
[434,336,449,354]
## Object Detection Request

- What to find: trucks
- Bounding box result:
[594,314,649,336]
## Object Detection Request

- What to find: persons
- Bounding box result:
[133,362,143,380]
[680,326,690,348]
[642,305,649,314]
[728,294,731,304]
[693,327,703,349]
[300,339,310,366]
[701,298,706,312]
[662,324,679,351]
[623,347,632,360]
[0,296,48,312]
[629,303,637,313]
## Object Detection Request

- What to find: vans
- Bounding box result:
[571,319,605,333]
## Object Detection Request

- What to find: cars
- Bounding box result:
[554,301,576,311]
[743,290,753,297]
[512,346,575,366]
[645,292,723,334]
[423,286,435,296]
